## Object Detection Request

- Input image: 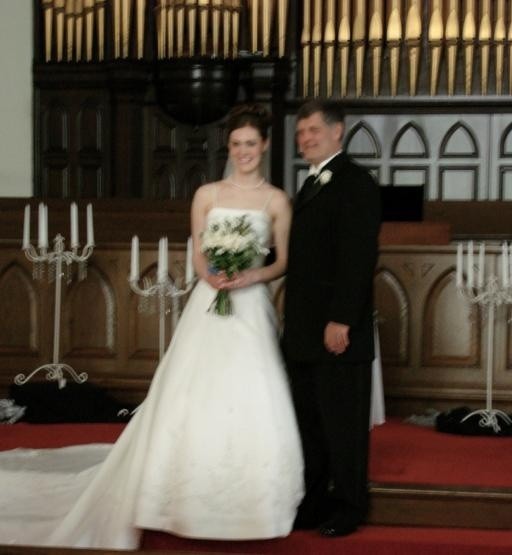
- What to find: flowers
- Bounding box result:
[199,211,272,316]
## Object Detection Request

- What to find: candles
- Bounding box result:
[22,200,96,250]
[129,233,198,286]
[456,239,511,290]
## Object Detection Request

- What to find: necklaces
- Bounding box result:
[223,176,271,191]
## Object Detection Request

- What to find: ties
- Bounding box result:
[298,175,315,204]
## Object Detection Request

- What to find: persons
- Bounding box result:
[0,102,306,551]
[279,98,381,535]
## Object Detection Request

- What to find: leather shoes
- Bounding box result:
[322,517,358,536]
